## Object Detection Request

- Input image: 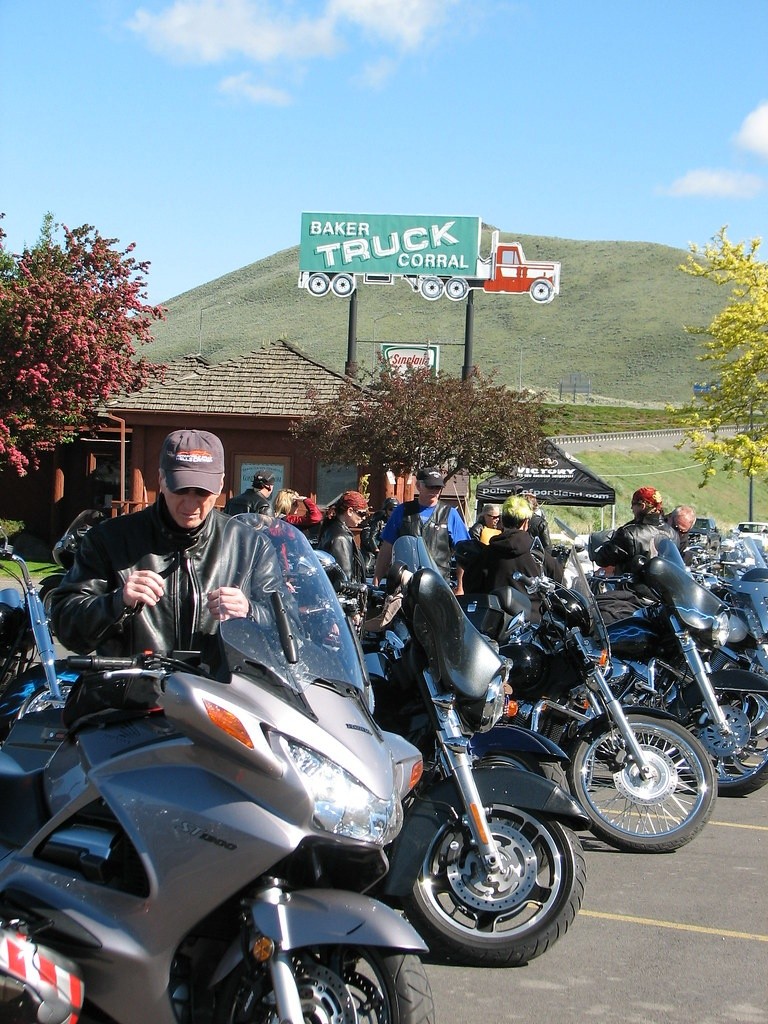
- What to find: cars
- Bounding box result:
[683,518,722,548]
[737,521,768,554]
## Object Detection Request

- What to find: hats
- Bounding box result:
[417,467,444,488]
[254,469,275,485]
[160,429,224,496]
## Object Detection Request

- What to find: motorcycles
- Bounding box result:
[535,514,768,797]
[247,535,597,964]
[305,539,719,855]
[37,510,129,619]
[0,510,437,1023]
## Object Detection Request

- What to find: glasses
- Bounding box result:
[487,513,501,520]
[387,507,397,512]
[672,517,689,535]
[631,500,642,507]
[166,488,215,498]
[352,508,367,519]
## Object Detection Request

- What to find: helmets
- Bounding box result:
[382,498,398,510]
[589,531,617,566]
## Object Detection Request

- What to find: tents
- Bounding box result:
[475,438,616,533]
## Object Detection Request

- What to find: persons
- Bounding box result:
[225,466,697,625]
[48,430,283,682]
